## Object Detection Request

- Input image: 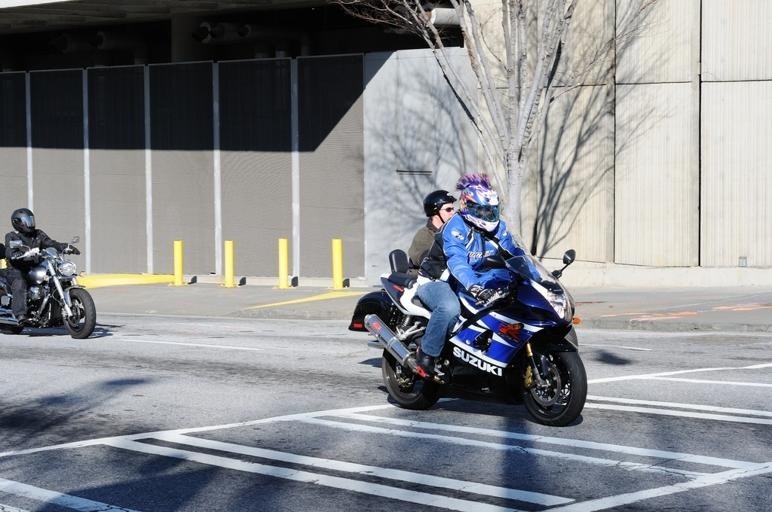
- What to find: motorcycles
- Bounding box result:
[1,232,98,340]
[350,244,589,430]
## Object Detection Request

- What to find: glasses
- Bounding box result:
[440,207,454,212]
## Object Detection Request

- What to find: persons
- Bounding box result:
[2,206,80,331]
[402,170,527,378]
[405,188,458,279]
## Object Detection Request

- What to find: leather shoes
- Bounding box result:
[417,348,435,375]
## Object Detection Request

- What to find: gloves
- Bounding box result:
[64,243,80,254]
[467,284,499,307]
[13,250,23,257]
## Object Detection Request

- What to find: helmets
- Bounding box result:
[459,185,499,232]
[11,208,35,233]
[423,190,457,216]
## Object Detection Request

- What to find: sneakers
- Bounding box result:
[17,314,26,323]
